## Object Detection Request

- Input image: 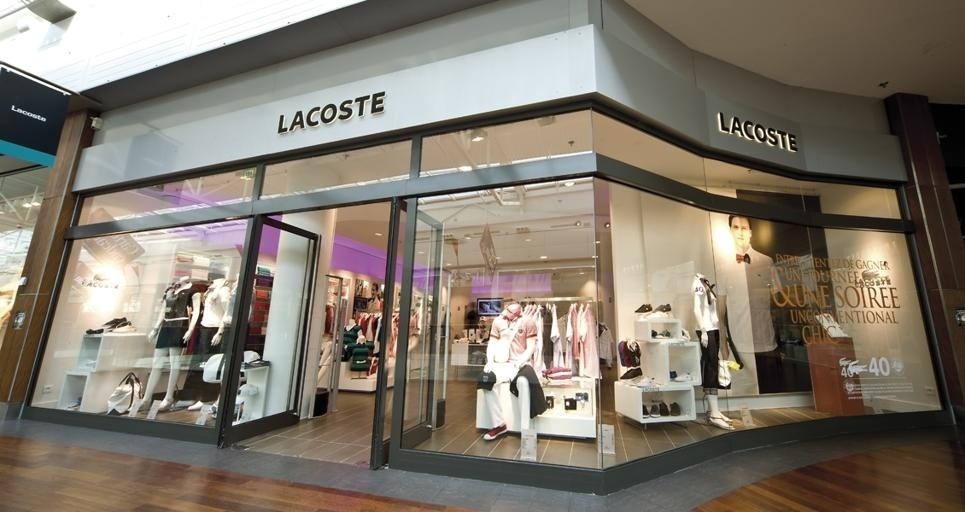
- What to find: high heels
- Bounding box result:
[706,411,735,431]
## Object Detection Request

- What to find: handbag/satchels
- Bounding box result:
[106,372,143,416]
[202,353,228,384]
[718,359,731,390]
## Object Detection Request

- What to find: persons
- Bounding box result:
[171,277,230,411]
[355,281,364,295]
[130,274,203,412]
[343,335,374,379]
[716,214,784,395]
[483,303,538,449]
[690,272,737,433]
[366,282,380,312]
[342,317,362,359]
[317,333,336,385]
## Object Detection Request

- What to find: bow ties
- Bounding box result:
[736,253,750,264]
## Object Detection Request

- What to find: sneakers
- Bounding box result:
[635,304,652,312]
[643,402,681,418]
[483,424,507,440]
[654,304,671,312]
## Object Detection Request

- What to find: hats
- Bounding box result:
[476,370,496,390]
[241,350,269,367]
[235,384,258,405]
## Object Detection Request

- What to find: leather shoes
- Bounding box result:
[104,317,131,332]
[619,367,642,379]
[86,328,104,334]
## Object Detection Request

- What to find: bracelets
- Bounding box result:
[217,332,223,337]
